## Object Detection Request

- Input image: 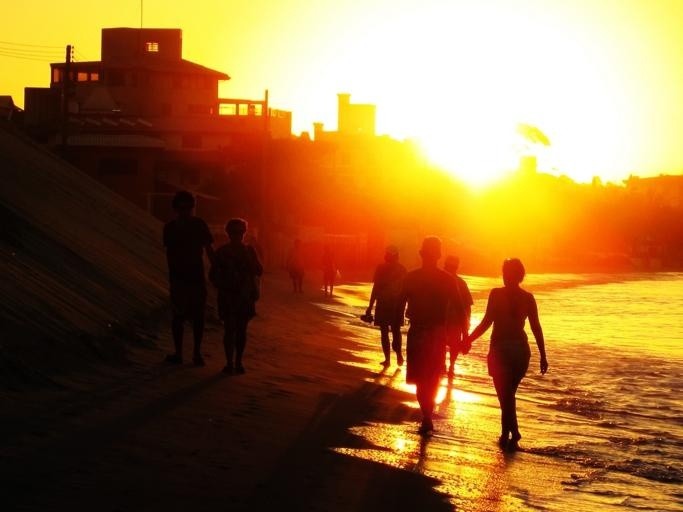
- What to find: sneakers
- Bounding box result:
[222,362,247,372]
[165,351,207,367]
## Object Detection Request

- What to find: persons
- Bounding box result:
[405,236,468,434]
[463,260,549,448]
[206,216,263,374]
[160,187,218,370]
[287,238,337,295]
[441,257,473,379]
[365,246,408,367]
